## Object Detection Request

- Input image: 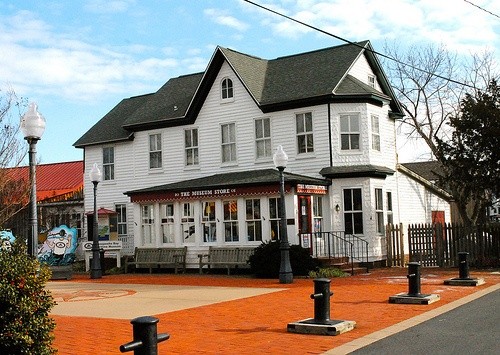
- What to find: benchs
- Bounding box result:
[198,246,254,275]
[124,246,187,275]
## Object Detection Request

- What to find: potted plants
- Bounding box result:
[39,249,77,280]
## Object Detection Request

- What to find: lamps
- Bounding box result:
[336,204,340,213]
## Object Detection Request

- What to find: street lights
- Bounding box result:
[20,100,47,262]
[272,144,293,283]
[88,162,104,279]
